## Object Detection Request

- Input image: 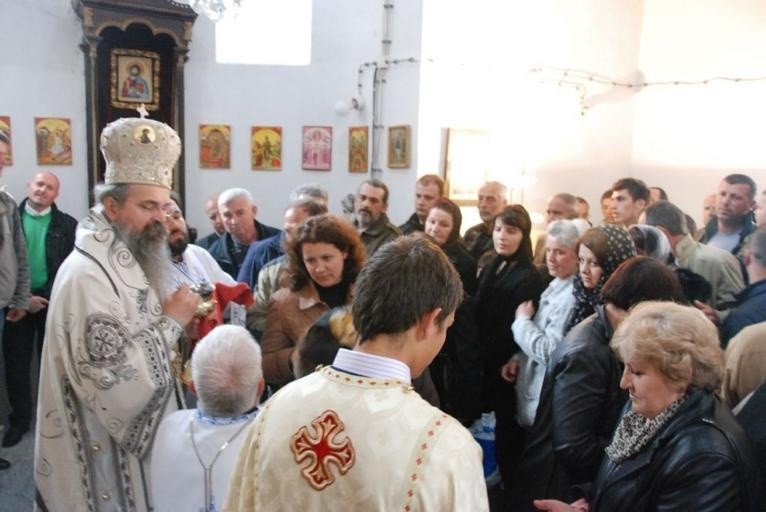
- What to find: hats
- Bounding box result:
[100,116,180,189]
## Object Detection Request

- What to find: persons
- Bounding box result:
[1,131,78,469]
[218,232,494,511]
[144,322,268,512]
[161,179,438,407]
[403,174,636,428]
[29,100,217,511]
[501,252,686,511]
[601,174,766,428]
[529,299,765,512]
[121,60,152,102]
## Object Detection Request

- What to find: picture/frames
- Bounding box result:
[384,123,412,171]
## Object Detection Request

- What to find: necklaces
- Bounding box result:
[189,413,252,511]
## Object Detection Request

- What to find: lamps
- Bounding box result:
[325,91,373,117]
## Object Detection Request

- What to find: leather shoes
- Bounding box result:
[2,425,30,447]
[0,459,9,469]
